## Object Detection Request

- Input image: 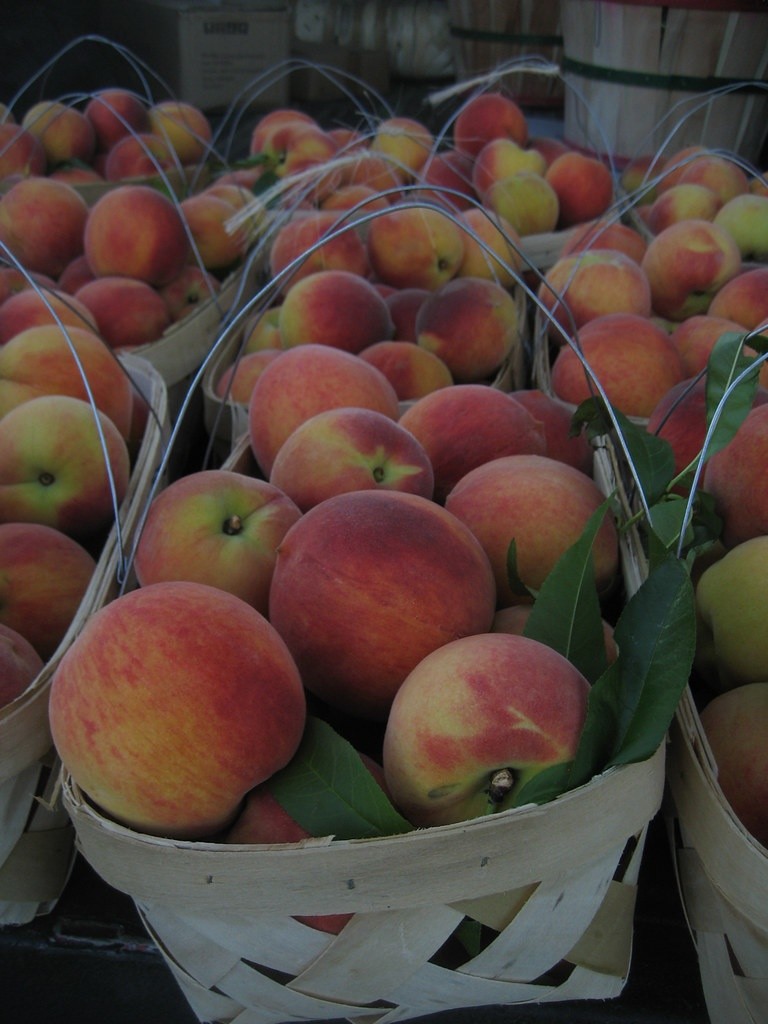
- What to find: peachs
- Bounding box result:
[1,90,768,933]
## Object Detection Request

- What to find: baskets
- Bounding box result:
[0,34,767,1024]
